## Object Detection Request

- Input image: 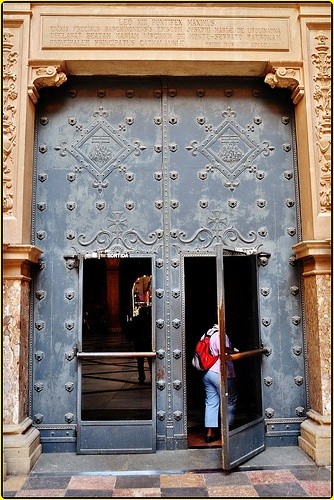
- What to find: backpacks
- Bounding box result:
[192,328,218,372]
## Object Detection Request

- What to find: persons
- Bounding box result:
[132,308,152,383]
[199,318,239,442]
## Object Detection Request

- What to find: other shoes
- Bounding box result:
[205,435,219,443]
